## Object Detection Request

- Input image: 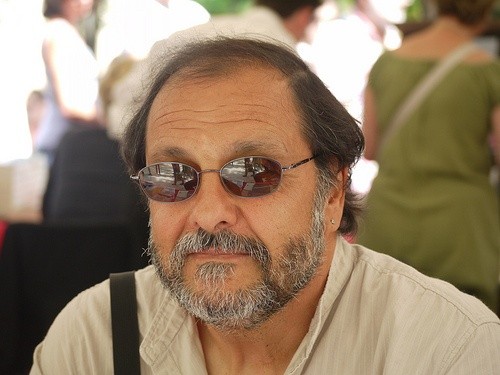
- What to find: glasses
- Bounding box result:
[129,152,323,204]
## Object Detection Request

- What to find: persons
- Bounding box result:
[361,0,500,315]
[296,0,415,196]
[28,0,99,156]
[30,24,500,375]
[95,0,327,140]
